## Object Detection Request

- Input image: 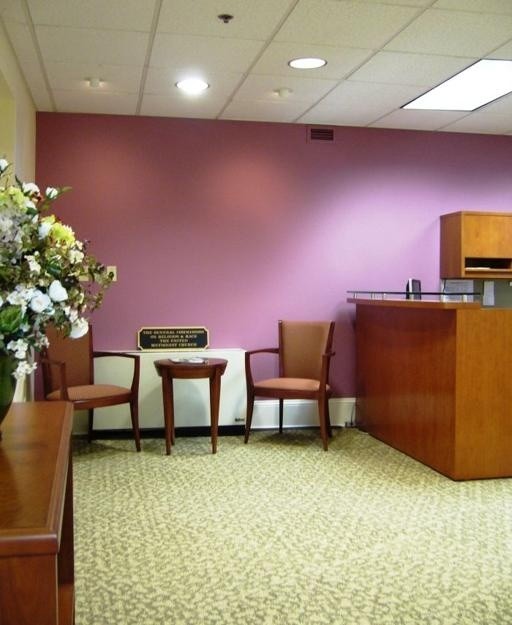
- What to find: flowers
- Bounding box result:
[0,154,117,376]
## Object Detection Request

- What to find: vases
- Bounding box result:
[0,352,17,441]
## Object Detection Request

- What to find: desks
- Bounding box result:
[155,358,227,455]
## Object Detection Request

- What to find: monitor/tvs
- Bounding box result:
[406,278,421,300]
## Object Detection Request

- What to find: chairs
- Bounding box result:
[37,318,143,453]
[242,319,338,450]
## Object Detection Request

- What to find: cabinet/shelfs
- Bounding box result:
[439,209,512,280]
[91,350,249,437]
[0,399,77,625]
